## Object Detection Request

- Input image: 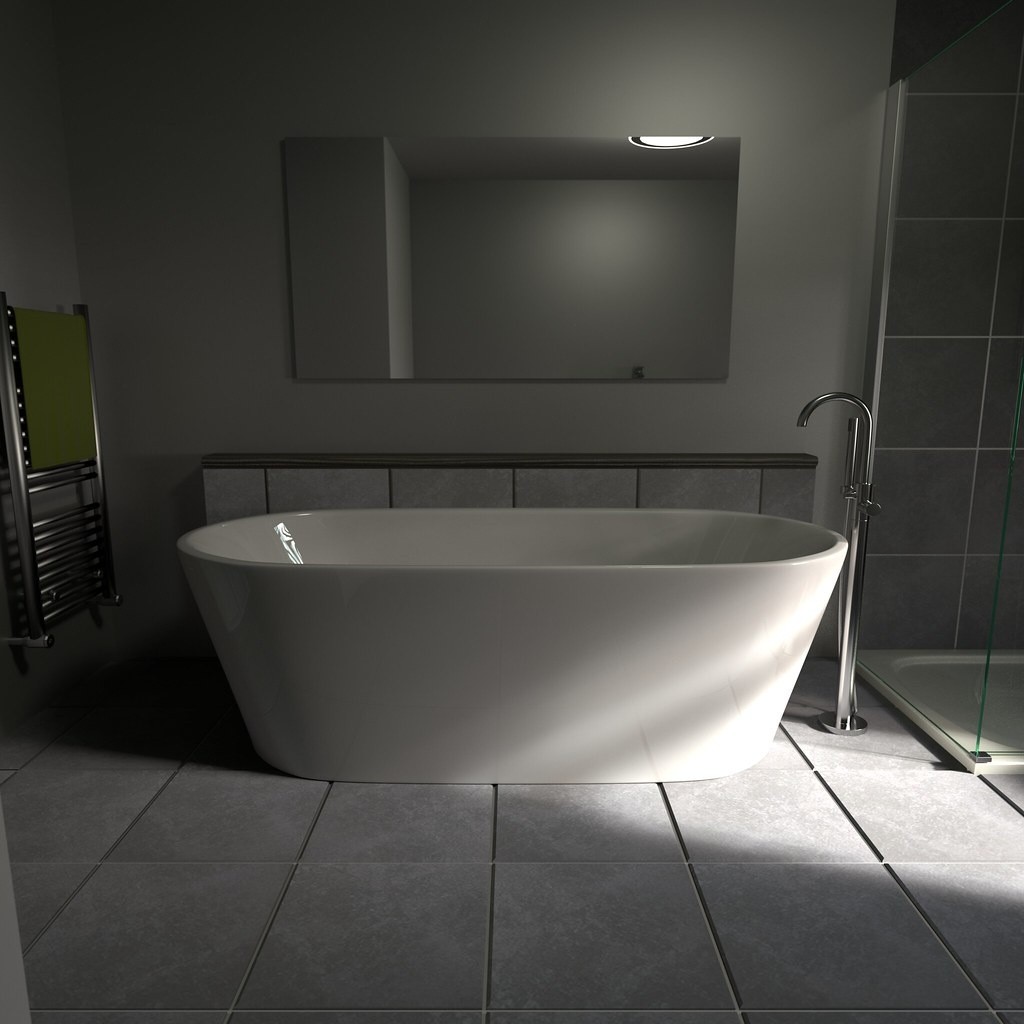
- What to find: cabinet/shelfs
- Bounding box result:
[201,449,819,523]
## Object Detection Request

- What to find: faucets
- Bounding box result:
[796,389,876,495]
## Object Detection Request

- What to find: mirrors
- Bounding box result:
[282,135,741,382]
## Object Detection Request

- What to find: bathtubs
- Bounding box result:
[180,507,852,784]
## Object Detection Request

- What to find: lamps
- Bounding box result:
[627,136,715,149]
